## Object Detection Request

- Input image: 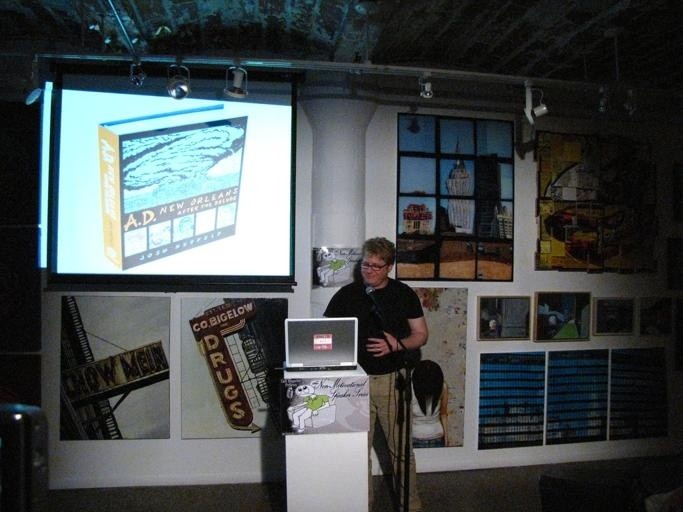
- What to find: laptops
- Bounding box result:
[285,317,358,371]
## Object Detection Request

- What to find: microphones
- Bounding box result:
[366,286,386,320]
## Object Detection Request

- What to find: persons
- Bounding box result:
[407,359,451,447]
[321,236,429,512]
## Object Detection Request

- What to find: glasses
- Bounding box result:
[360,262,389,272]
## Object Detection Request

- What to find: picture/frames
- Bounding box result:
[475,291,674,344]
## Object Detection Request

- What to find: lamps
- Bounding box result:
[128,59,251,101]
[418,77,435,99]
[18,66,43,106]
[524,80,550,126]
[598,89,611,113]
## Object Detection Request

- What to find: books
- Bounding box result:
[95,101,253,274]
